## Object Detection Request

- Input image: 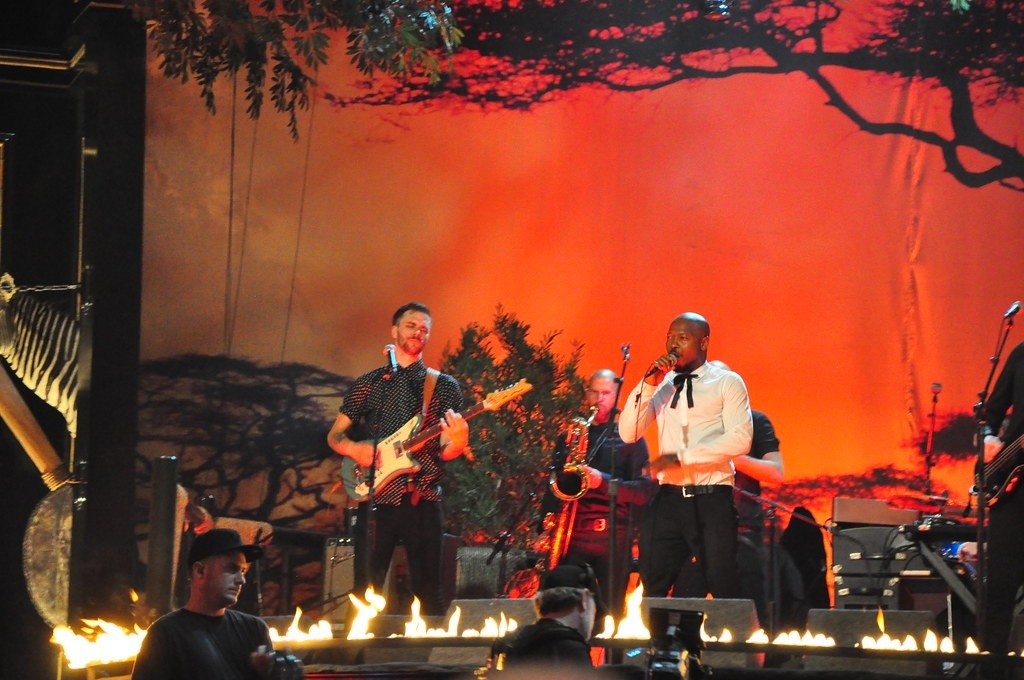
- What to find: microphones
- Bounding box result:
[383,344,399,372]
[931,383,941,402]
[1003,301,1023,318]
[645,354,677,377]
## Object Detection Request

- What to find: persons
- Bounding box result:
[619,312,783,657]
[535,369,651,638]
[492,565,611,680]
[977,342,1024,656]
[131,528,275,680]
[327,302,469,639]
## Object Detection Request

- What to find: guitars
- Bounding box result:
[342,378,533,502]
[974,434,1024,494]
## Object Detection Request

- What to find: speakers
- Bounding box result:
[640,596,761,642]
[442,599,538,638]
[807,608,939,650]
[322,537,355,617]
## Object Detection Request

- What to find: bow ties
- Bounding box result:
[670,372,698,409]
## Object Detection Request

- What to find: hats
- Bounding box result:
[185,529,262,563]
[537,562,609,621]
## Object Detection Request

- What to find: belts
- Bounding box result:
[666,484,725,499]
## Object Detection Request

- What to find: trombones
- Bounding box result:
[549,404,599,572]
